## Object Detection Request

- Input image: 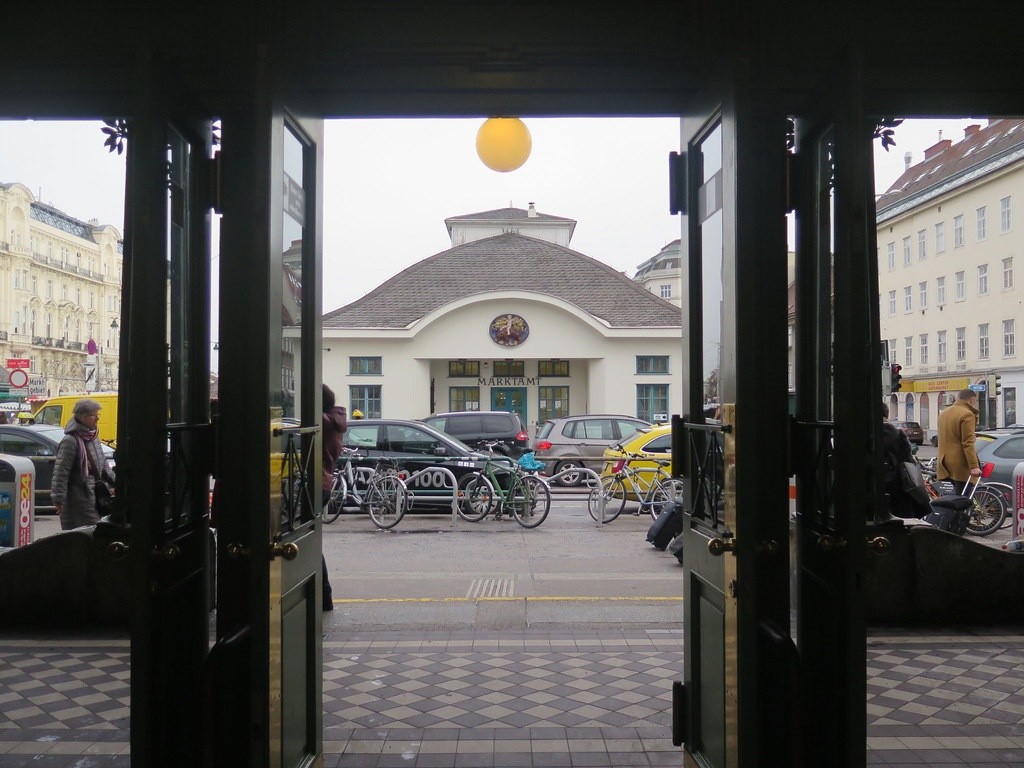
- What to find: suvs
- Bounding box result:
[533,414,655,486]
[885,421,924,446]
[419,412,528,459]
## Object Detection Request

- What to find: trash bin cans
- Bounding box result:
[1013,462,1024,538]
[0,454,35,548]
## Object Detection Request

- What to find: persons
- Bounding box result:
[300,383,347,613]
[936,389,985,511]
[881,402,914,520]
[49,399,116,531]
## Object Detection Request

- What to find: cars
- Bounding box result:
[923,427,1023,513]
[599,422,672,502]
[1,421,117,511]
[930,434,938,447]
[284,417,537,514]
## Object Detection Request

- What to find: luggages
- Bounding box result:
[670,532,684,563]
[923,475,982,534]
[646,501,686,552]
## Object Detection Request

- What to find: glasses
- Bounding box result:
[88,412,102,417]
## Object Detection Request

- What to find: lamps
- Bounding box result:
[475,118,532,173]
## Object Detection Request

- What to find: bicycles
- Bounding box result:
[319,447,408,529]
[457,441,551,529]
[587,444,683,524]
[911,444,1013,536]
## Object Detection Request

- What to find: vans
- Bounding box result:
[16,395,117,449]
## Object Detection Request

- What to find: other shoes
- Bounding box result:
[321,588,336,611]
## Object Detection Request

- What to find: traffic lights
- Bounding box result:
[891,364,903,393]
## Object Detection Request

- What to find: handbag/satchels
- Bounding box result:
[94,477,116,518]
[889,430,934,520]
